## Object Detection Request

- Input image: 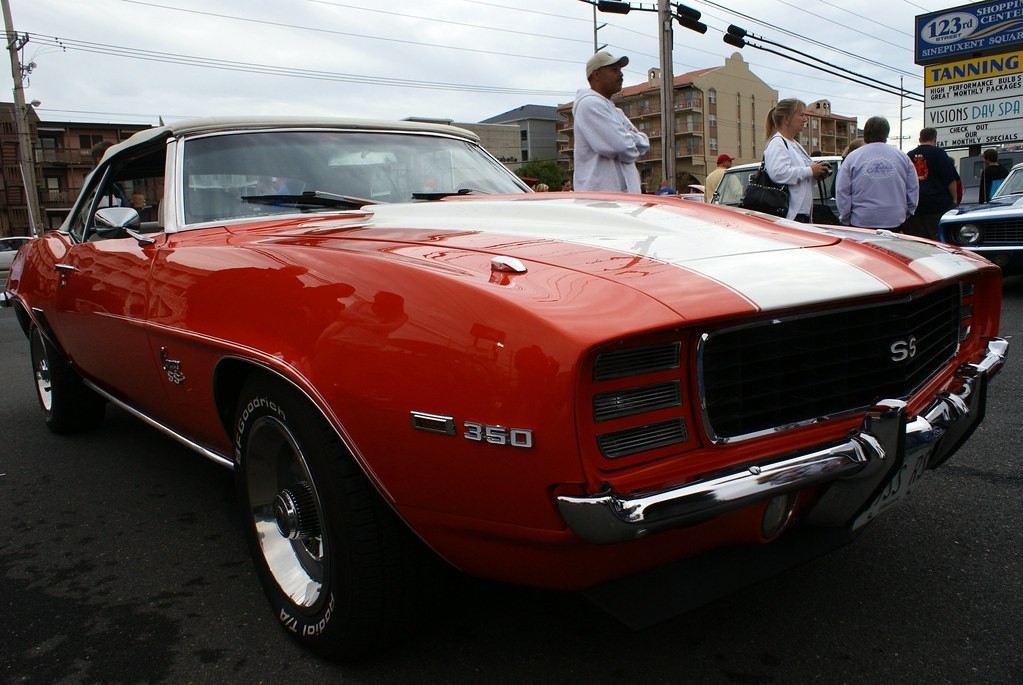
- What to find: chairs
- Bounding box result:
[158,186,235,232]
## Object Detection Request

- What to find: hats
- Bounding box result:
[586,51,629,82]
[717,154,735,164]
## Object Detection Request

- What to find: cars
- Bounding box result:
[0,237,39,271]
[7,114,1011,671]
[939,163,1023,275]
[708,154,844,225]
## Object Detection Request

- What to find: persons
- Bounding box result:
[908,128,963,240]
[836,117,920,233]
[763,97,829,223]
[842,138,867,157]
[656,181,677,195]
[703,153,744,203]
[811,151,822,157]
[561,179,573,192]
[571,51,650,194]
[537,184,549,192]
[979,149,1009,204]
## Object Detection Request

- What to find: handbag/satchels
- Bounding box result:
[737,136,791,218]
